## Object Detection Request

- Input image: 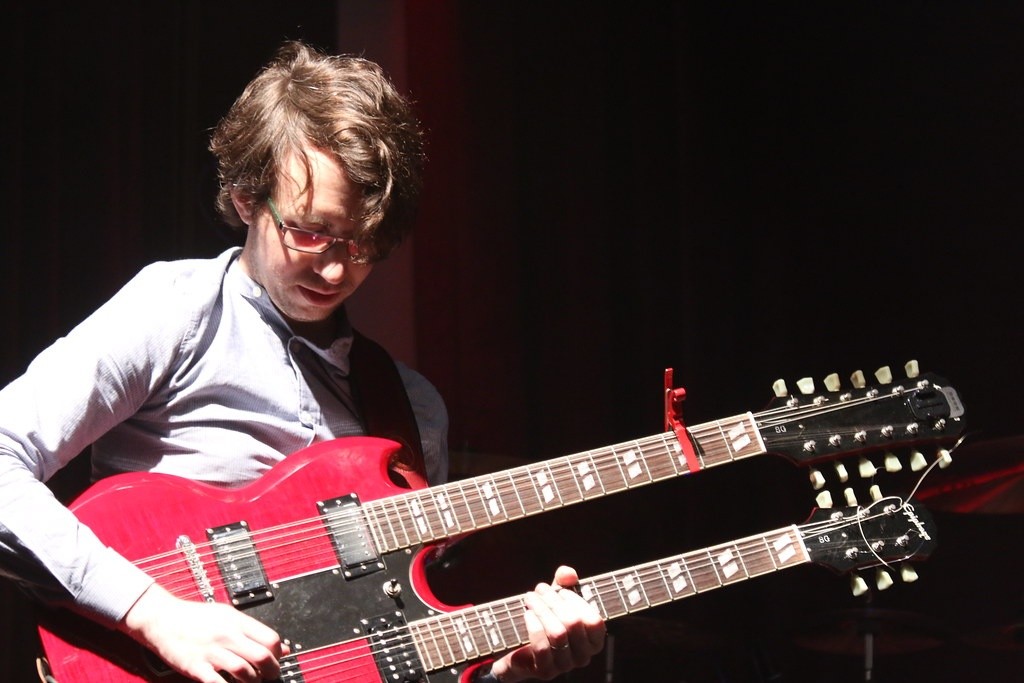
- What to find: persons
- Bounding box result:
[0,40,606,683]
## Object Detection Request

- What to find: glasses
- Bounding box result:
[266,194,386,264]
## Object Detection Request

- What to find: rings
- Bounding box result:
[550,642,570,651]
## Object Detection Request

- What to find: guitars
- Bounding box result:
[31,356,972,683]
